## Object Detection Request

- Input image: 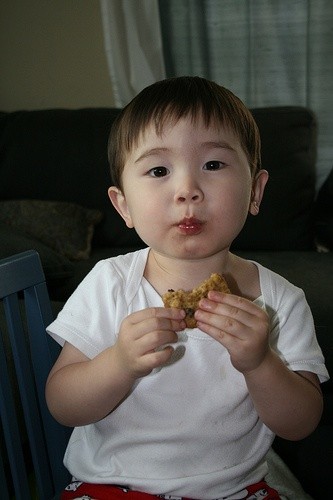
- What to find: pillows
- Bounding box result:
[1,200,99,261]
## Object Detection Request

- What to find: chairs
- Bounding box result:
[0,250,72,499]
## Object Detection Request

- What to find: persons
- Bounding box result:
[45,75,331,500]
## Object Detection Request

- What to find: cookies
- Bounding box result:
[162,273,231,329]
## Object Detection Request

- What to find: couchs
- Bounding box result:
[0,106,333,347]
